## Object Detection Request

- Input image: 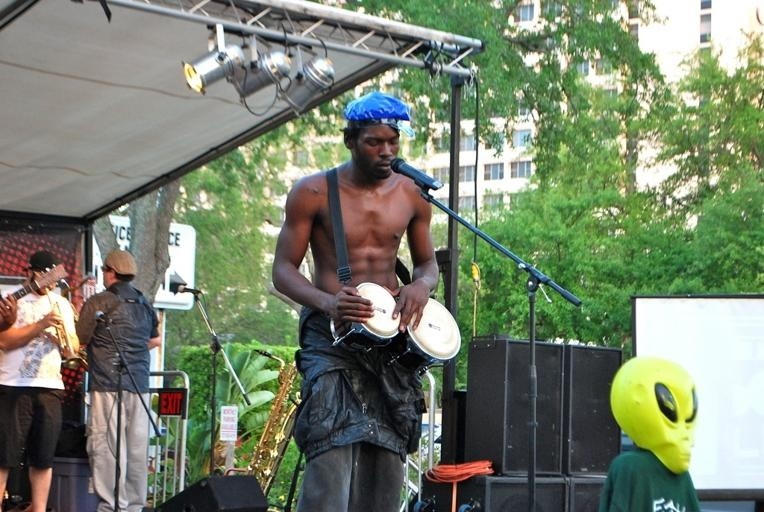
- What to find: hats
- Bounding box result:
[344,92,410,121]
[22,250,58,273]
[104,249,138,277]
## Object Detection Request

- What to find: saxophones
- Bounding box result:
[245,349,303,498]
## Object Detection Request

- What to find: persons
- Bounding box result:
[599,358,701,512]
[272,91,439,512]
[1,251,82,511]
[76,250,163,512]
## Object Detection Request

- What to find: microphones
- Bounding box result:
[391,158,444,191]
[171,283,201,296]
[96,310,118,326]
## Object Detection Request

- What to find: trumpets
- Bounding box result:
[45,287,83,370]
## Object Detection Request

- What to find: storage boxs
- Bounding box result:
[48,457,102,512]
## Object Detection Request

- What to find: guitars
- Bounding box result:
[0,263,69,306]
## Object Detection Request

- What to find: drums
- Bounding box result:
[330,281,401,353]
[387,297,461,374]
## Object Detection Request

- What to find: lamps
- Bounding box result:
[180,20,336,112]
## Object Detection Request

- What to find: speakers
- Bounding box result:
[152,473,268,512]
[454,334,623,512]
